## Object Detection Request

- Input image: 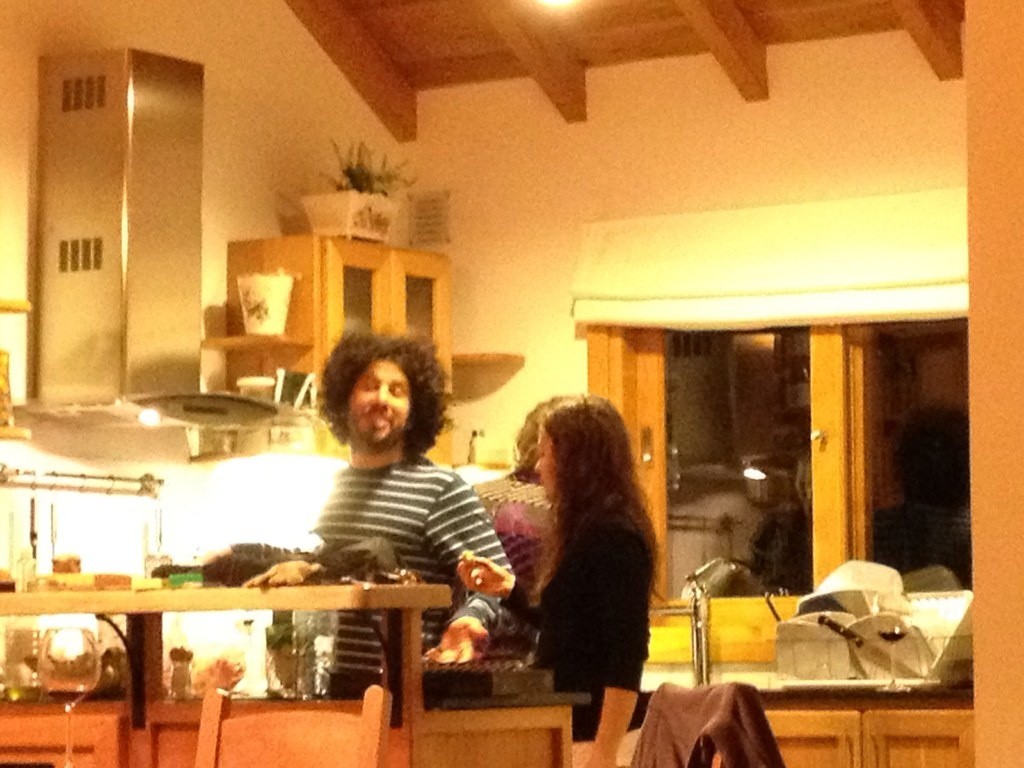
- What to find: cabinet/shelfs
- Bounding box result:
[765,704,974,768]
[199,236,454,466]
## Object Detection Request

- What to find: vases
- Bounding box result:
[235,271,296,337]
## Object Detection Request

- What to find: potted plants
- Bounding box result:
[298,137,414,243]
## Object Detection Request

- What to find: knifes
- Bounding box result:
[818,616,924,679]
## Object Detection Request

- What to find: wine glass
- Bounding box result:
[37,628,102,768]
[869,594,913,692]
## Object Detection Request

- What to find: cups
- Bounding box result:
[238,276,293,334]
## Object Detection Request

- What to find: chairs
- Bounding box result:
[0,684,393,768]
[629,682,786,768]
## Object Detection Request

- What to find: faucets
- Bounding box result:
[649,554,782,688]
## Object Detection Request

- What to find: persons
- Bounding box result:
[526,392,662,768]
[474,397,584,651]
[198,321,519,700]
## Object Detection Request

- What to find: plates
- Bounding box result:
[775,611,856,679]
[847,613,928,678]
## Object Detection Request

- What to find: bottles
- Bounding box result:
[170,649,192,698]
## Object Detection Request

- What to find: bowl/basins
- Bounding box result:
[798,561,904,611]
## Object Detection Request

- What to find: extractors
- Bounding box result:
[15,47,277,429]
